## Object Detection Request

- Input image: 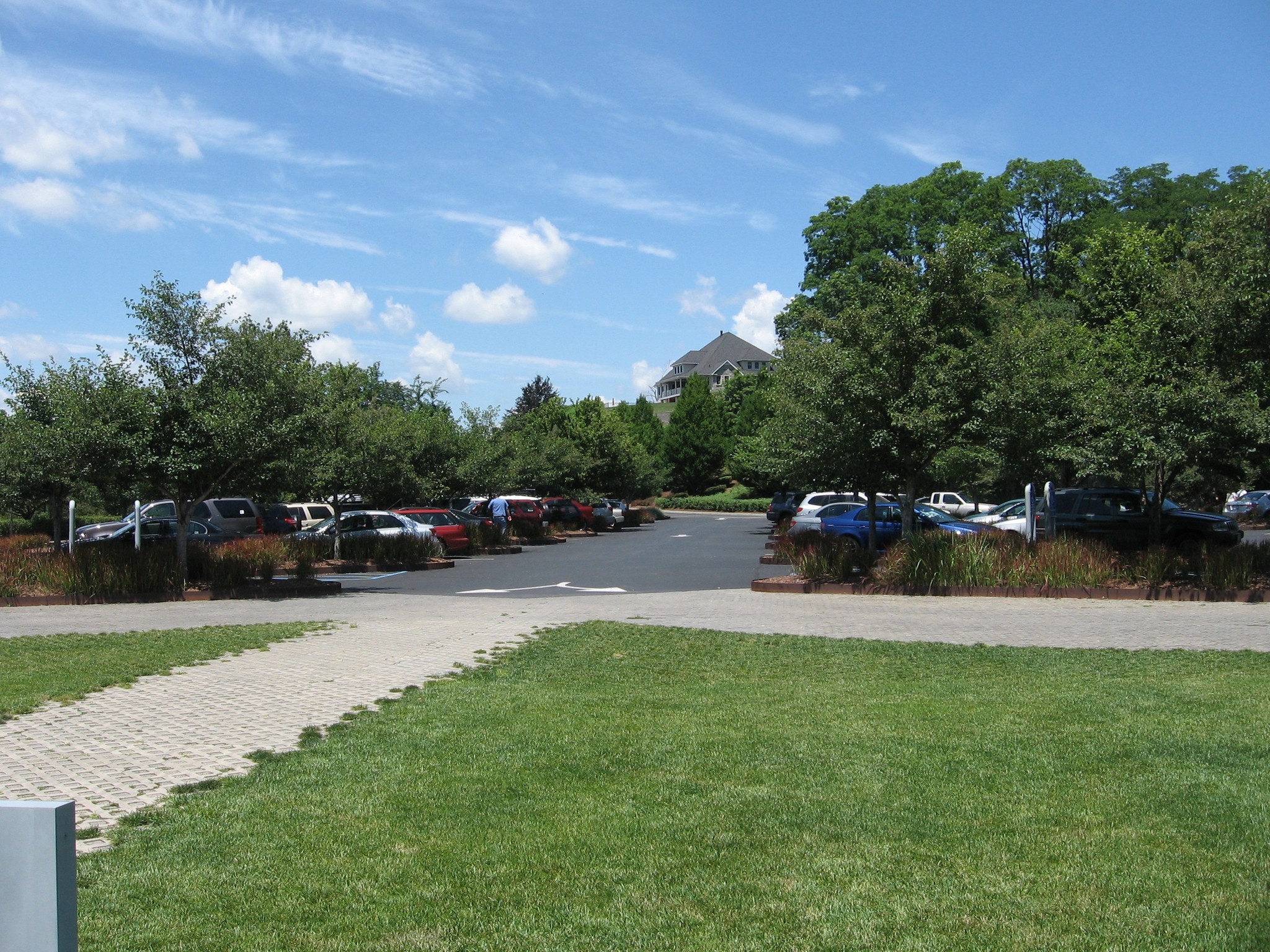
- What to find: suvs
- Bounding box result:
[1035,486,1245,556]
[796,491,904,517]
[765,491,807,529]
[74,495,267,540]
[281,502,336,531]
[255,503,302,536]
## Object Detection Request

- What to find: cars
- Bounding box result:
[585,498,616,531]
[820,502,1010,561]
[447,508,496,545]
[388,508,471,557]
[1221,490,1270,522]
[282,510,441,568]
[964,484,1043,541]
[62,515,251,546]
[463,496,549,535]
[605,499,629,526]
[541,497,595,530]
[784,502,868,552]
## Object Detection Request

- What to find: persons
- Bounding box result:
[488,494,512,534]
[1226,485,1247,503]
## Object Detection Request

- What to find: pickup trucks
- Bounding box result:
[922,492,1000,519]
